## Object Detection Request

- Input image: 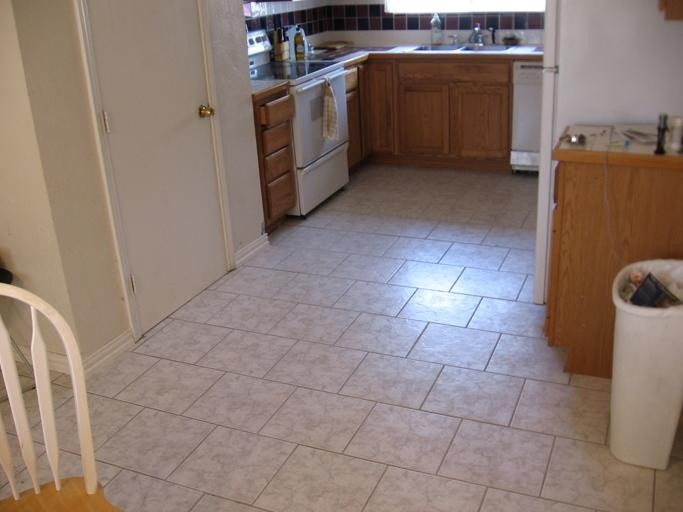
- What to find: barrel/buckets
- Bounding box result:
[286,22,308,59]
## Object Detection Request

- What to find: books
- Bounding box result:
[315,41,352,49]
[629,271,683,307]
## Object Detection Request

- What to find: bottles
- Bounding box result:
[472,22,481,46]
[293,27,304,60]
[296,62,305,77]
[652,111,682,154]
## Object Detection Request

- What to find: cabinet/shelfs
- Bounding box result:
[362,46,398,163]
[388,49,513,175]
[541,118,681,382]
[248,76,297,233]
[346,55,368,176]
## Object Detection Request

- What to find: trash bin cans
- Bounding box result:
[608,258,683,471]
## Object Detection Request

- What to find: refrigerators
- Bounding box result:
[531,1,682,305]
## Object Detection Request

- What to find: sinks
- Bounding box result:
[461,43,518,52]
[413,43,464,51]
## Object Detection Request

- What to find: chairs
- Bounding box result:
[0,282,130,512]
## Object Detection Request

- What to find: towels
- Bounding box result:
[318,75,341,144]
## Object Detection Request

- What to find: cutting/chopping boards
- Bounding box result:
[315,41,345,50]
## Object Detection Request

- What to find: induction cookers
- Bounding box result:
[246,63,346,81]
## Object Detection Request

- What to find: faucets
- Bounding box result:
[467,22,481,43]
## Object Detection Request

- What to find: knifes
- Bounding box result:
[276,28,286,43]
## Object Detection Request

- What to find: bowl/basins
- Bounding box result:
[503,39,518,45]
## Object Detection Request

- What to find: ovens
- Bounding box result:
[286,66,349,218]
